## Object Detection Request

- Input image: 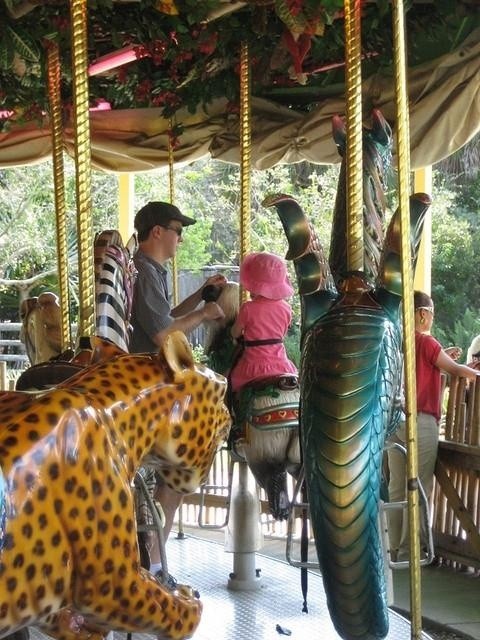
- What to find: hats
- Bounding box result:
[134,201,197,230]
[238,251,294,302]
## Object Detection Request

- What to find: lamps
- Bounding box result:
[88,30,179,78]
[0,97,112,120]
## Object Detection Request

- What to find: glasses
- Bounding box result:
[159,223,184,239]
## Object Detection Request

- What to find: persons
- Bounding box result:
[385,290,480,568]
[127,201,227,599]
[226,253,298,448]
